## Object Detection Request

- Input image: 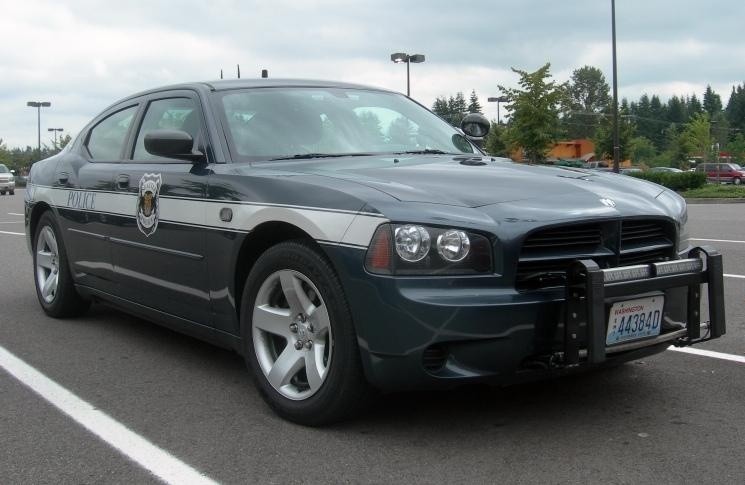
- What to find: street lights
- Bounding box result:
[48,127,64,156]
[488,95,509,128]
[27,100,51,164]
[389,52,429,146]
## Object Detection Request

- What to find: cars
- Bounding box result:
[597,154,745,188]
[0,162,17,197]
[23,64,730,432]
